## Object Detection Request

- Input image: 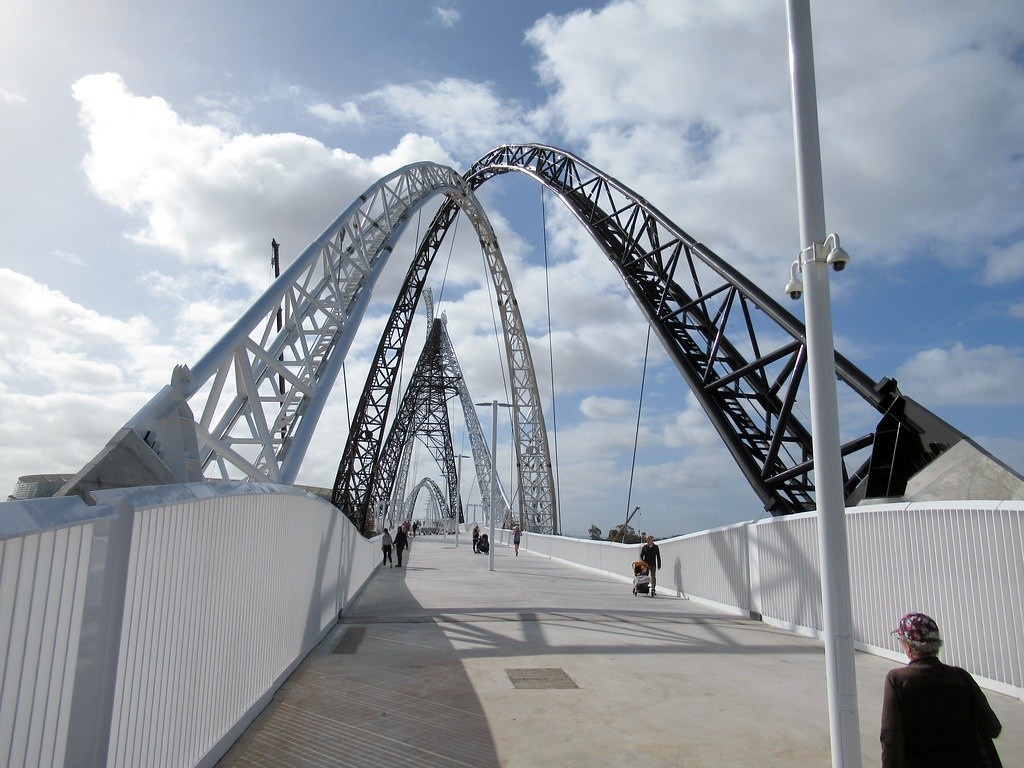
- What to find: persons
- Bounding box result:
[636,535,661,595]
[412,522,417,537]
[879,611,1003,768]
[381,527,394,568]
[472,525,482,554]
[394,522,408,567]
[513,526,522,556]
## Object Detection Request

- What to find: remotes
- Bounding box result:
[827,247,849,272]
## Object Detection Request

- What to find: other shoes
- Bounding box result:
[651,589,656,595]
[516,552,518,556]
[395,565,401,567]
[382,565,386,568]
[478,551,480,553]
[474,552,477,553]
[414,535,415,537]
[390,563,392,568]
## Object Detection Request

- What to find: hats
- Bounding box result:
[890,613,939,642]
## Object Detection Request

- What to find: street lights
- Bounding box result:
[475,400,515,571]
[452,453,470,547]
[784,232,862,768]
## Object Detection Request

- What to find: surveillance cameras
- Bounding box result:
[785,280,803,300]
